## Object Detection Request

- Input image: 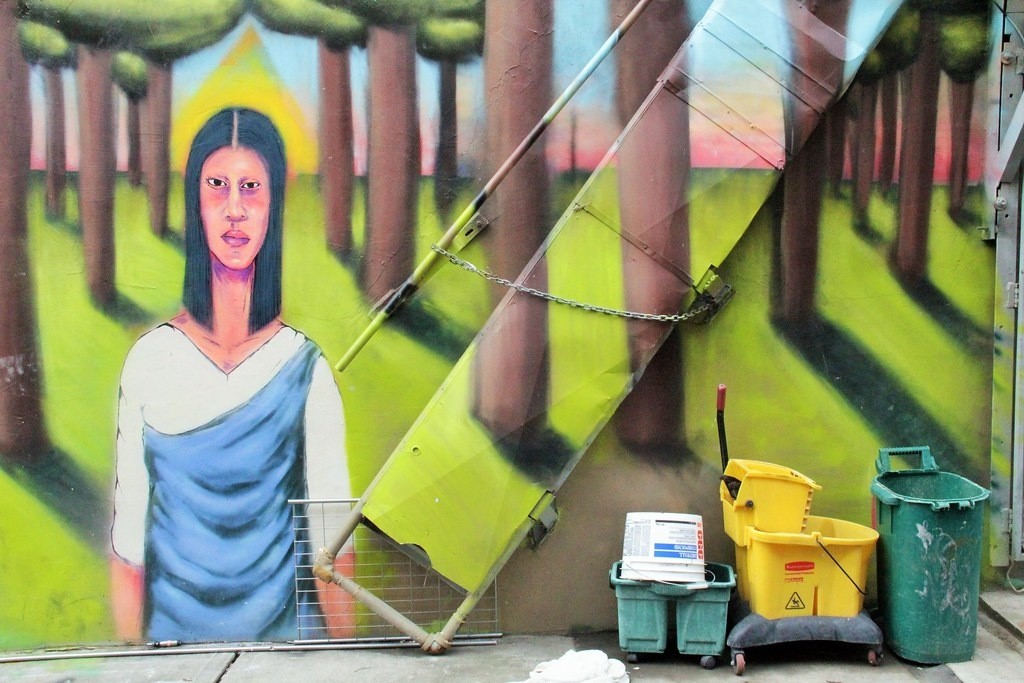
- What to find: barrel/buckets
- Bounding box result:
[617,511,715,589]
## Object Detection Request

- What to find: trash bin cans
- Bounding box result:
[871,446,991,664]
